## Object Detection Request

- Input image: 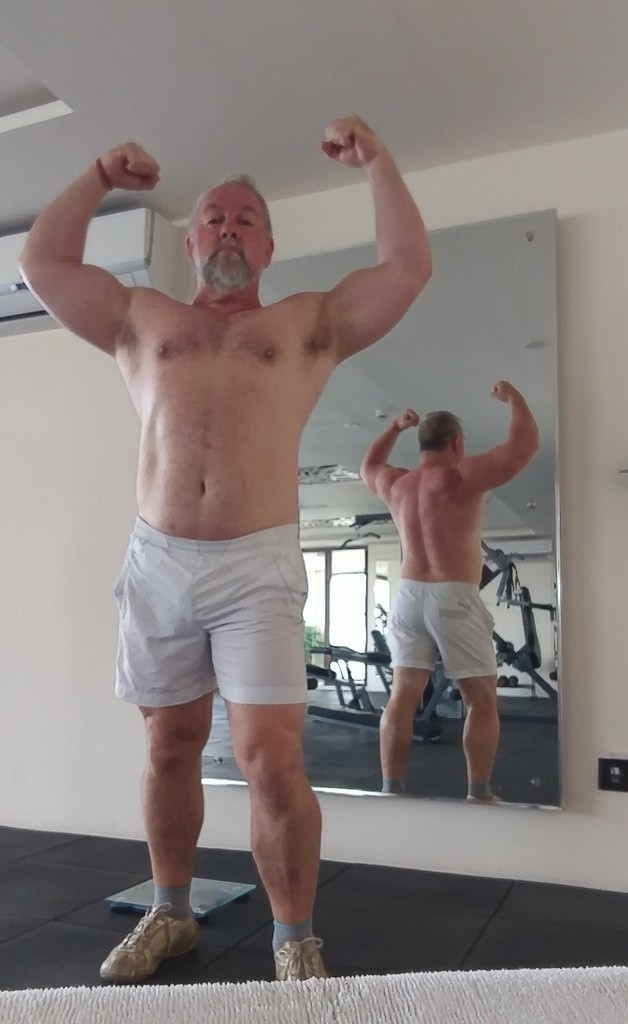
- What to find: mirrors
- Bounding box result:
[200,206,561,812]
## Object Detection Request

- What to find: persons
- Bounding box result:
[360,381,540,802]
[14,116,434,983]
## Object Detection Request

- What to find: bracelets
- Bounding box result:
[95,159,114,191]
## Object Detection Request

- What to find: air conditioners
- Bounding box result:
[0,207,188,339]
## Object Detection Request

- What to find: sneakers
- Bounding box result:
[466,793,503,802]
[99,901,201,981]
[274,936,329,982]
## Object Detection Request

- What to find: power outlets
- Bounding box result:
[598,758,628,792]
